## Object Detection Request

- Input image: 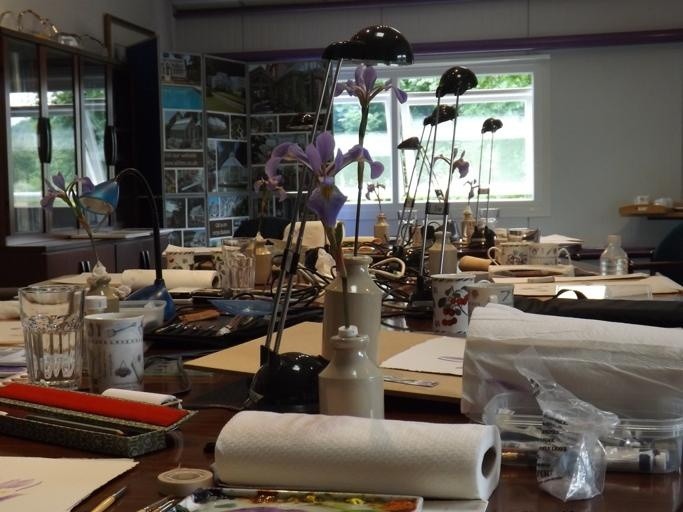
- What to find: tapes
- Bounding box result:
[157,468,212,498]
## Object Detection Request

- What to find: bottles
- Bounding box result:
[600,235,628,275]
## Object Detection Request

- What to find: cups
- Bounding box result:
[480,208,501,229]
[167,252,193,269]
[222,238,255,293]
[83,313,145,397]
[487,241,530,275]
[17,284,85,392]
[530,243,571,266]
[464,282,514,330]
[429,272,477,337]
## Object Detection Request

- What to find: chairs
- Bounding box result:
[649,224,683,286]
[282,221,347,249]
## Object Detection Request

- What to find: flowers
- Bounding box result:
[366,181,385,212]
[330,64,407,255]
[255,175,287,232]
[464,180,479,205]
[430,148,469,232]
[262,130,385,328]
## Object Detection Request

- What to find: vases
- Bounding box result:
[322,255,382,365]
[374,216,389,241]
[461,214,476,238]
[246,241,271,285]
[86,277,119,316]
[318,335,384,419]
[429,231,457,274]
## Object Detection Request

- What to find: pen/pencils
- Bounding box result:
[137,494,177,512]
[90,485,128,512]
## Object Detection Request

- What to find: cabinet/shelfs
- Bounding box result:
[2,230,159,286]
[0,25,117,246]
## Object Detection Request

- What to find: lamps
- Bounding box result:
[420,66,479,274]
[475,118,504,235]
[395,106,458,248]
[260,25,415,352]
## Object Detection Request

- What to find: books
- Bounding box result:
[70,230,153,239]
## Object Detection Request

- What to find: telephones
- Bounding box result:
[104,125,119,167]
[37,116,52,164]
